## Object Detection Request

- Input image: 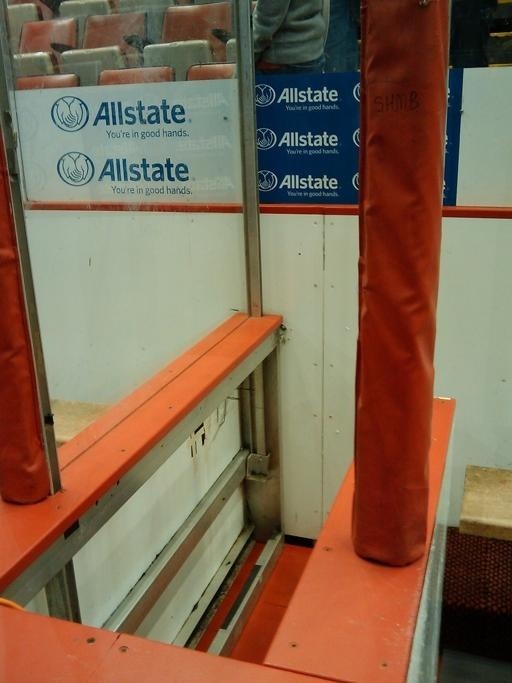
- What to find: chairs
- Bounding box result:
[1,0,258,89]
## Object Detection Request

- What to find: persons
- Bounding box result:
[251,0,331,72]
